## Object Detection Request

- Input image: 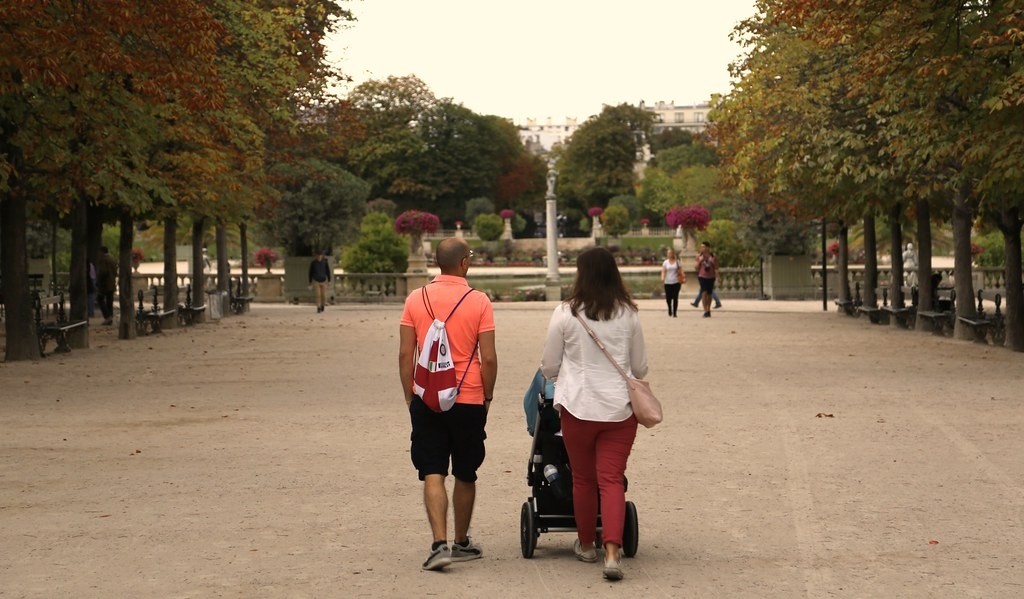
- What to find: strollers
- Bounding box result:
[521,366,638,558]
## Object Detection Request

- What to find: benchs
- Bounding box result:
[834,281,863,318]
[229,278,254,314]
[958,289,1006,345]
[858,288,890,325]
[882,287,917,329]
[135,286,176,334]
[919,290,955,336]
[34,291,87,354]
[177,285,206,326]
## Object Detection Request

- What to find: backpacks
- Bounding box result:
[414,285,478,411]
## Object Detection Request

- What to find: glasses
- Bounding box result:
[461,250,474,266]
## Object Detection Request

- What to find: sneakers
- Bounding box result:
[422,545,452,570]
[451,537,482,562]
[573,539,597,562]
[604,559,623,579]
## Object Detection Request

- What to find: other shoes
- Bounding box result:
[673,309,676,317]
[669,308,672,316]
[713,303,721,308]
[690,303,699,307]
[703,311,710,317]
[317,307,320,313]
[321,305,324,311]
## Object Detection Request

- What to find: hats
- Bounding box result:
[314,249,324,255]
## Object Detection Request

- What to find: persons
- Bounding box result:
[540,248,648,580]
[546,163,560,195]
[399,238,498,569]
[691,286,721,308]
[695,241,718,318]
[202,247,211,274]
[902,242,918,287]
[308,250,331,312]
[86,259,94,317]
[661,246,686,317]
[95,246,116,325]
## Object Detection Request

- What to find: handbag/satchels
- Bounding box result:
[677,261,685,282]
[626,376,663,428]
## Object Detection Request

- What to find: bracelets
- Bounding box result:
[484,396,493,401]
